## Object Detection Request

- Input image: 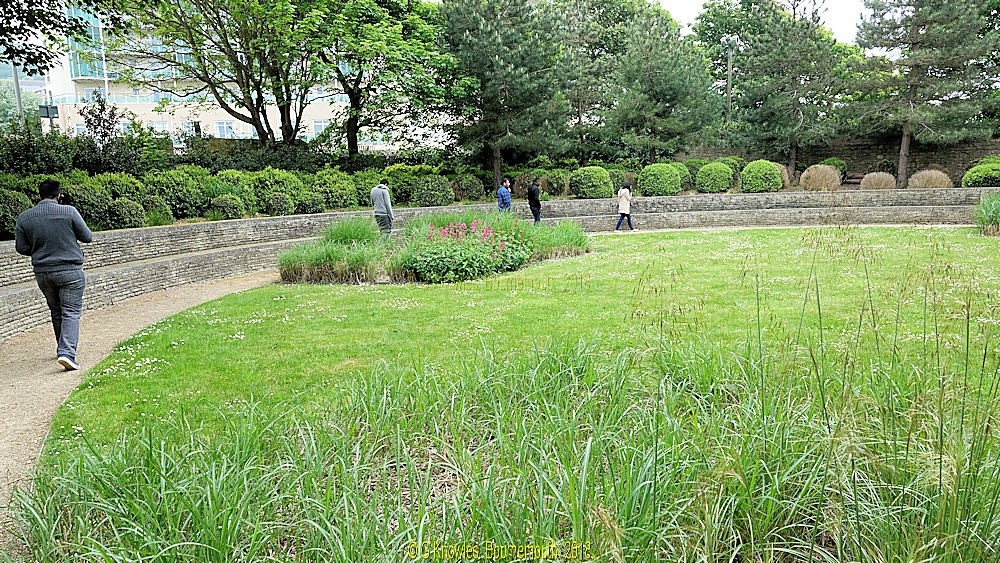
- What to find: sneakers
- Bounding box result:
[57,355,81,370]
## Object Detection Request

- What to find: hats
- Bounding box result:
[623,182,632,186]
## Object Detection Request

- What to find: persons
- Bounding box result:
[370,179,394,234]
[613,183,640,234]
[497,177,512,212]
[17,179,92,370]
[527,177,543,226]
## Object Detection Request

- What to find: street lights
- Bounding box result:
[719,34,746,123]
